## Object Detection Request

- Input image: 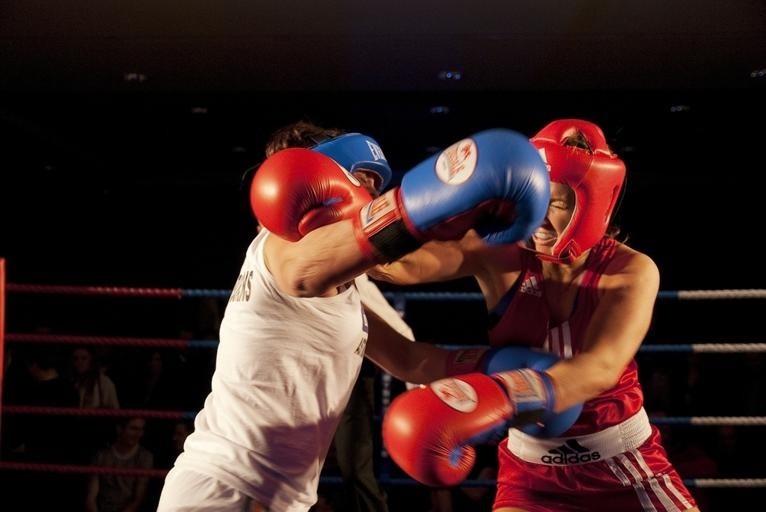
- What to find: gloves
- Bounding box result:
[383,367,555,487]
[450,345,582,441]
[248,149,373,242]
[355,129,549,260]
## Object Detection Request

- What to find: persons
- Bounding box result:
[429,465,496,510]
[336,358,388,510]
[251,118,701,512]
[647,402,718,501]
[1,344,195,510]
[156,121,551,512]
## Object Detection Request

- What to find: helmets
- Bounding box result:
[314,131,392,194]
[529,118,628,266]
